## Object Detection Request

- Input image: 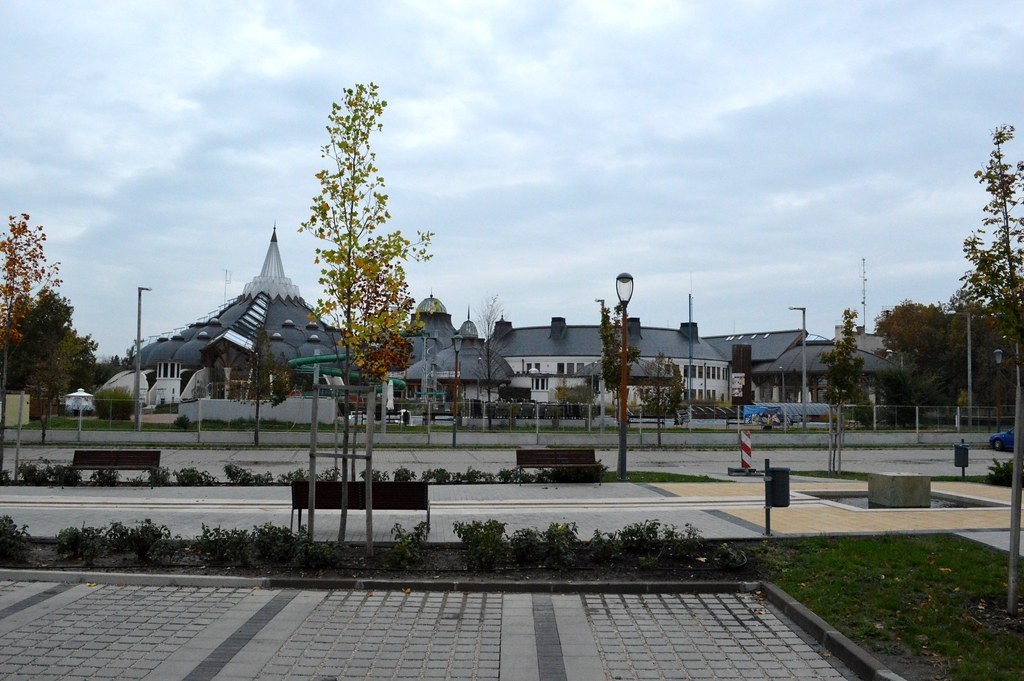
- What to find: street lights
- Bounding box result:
[133,286,152,431]
[992,348,1003,433]
[451,335,463,450]
[615,273,635,481]
[787,305,807,432]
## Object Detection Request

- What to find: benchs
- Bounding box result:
[515,449,603,487]
[61,450,161,489]
[289,481,431,540]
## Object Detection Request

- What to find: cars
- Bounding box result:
[989,426,1014,451]
